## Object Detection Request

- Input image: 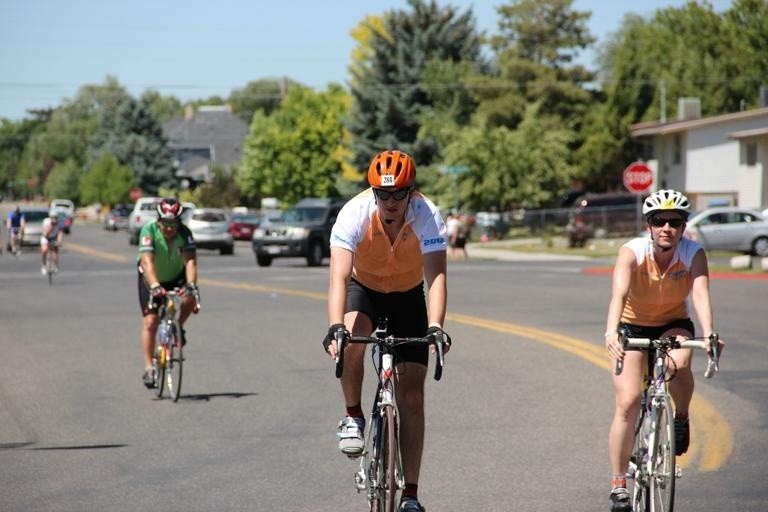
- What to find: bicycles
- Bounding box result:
[334,326,446,512]
[41,240,58,285]
[146,284,200,401]
[615,323,720,512]
[6,227,26,256]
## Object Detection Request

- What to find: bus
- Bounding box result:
[49,199,75,231]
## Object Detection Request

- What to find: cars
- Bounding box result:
[104,195,263,256]
[13,205,58,245]
[640,206,768,257]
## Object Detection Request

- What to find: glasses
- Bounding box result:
[374,188,408,200]
[162,221,178,228]
[649,216,682,228]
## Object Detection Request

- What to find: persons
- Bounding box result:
[39,210,64,276]
[134,197,202,387]
[451,216,471,262]
[6,206,26,255]
[445,211,459,248]
[604,189,724,512]
[322,150,452,511]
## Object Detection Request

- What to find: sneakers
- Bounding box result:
[337,417,365,454]
[144,368,156,387]
[673,412,689,455]
[397,498,425,512]
[173,324,186,346]
[609,487,631,511]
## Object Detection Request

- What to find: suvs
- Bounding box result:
[252,197,348,268]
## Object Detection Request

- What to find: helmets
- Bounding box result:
[369,151,416,188]
[159,197,183,220]
[642,189,691,216]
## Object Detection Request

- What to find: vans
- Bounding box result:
[567,190,653,237]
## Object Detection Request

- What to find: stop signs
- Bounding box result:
[622,161,653,195]
